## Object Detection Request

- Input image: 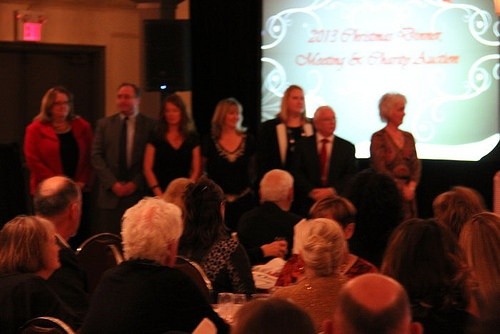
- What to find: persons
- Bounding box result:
[295,109,357,215]
[90,82,153,235]
[0,168,500,334]
[371,93,421,220]
[256,85,317,168]
[26,87,93,247]
[143,95,201,197]
[199,97,258,234]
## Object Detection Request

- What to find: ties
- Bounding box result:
[117,116,129,175]
[319,138,329,184]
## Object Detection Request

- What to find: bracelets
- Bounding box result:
[148,185,161,192]
[408,186,415,190]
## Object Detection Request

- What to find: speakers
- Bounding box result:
[142,19,191,92]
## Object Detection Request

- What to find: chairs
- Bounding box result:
[16,315,77,334]
[175,256,214,304]
[77,233,123,290]
